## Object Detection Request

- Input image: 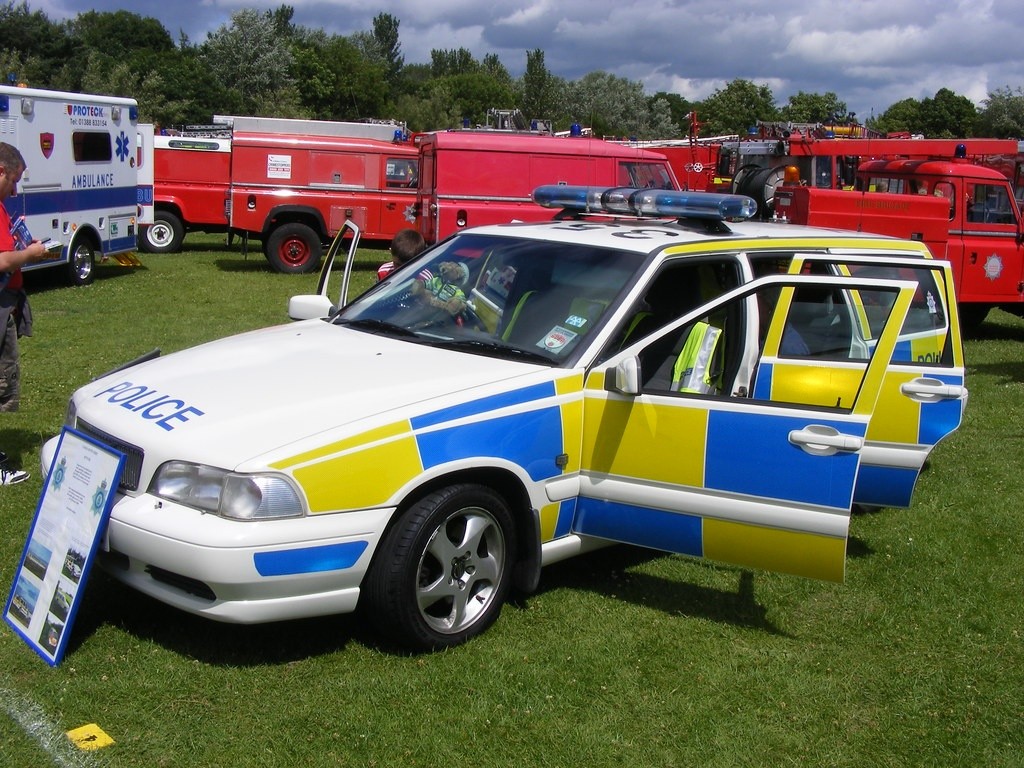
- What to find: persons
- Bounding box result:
[876,182,890,193]
[378,230,432,283]
[0,143,50,485]
[758,298,811,357]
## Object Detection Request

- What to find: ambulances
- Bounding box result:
[0,72,156,289]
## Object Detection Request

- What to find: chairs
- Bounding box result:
[500,289,657,370]
[641,283,739,395]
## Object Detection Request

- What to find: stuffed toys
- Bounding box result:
[411,262,470,316]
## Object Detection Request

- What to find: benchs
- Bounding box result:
[789,301,931,355]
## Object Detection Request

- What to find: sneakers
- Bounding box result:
[0,469,30,485]
[0,452,8,463]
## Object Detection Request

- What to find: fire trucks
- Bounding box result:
[137,107,690,275]
[599,119,1024,334]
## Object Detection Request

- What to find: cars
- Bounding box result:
[67,561,81,578]
[39,185,969,656]
[54,589,69,613]
[12,593,28,618]
[48,627,59,648]
